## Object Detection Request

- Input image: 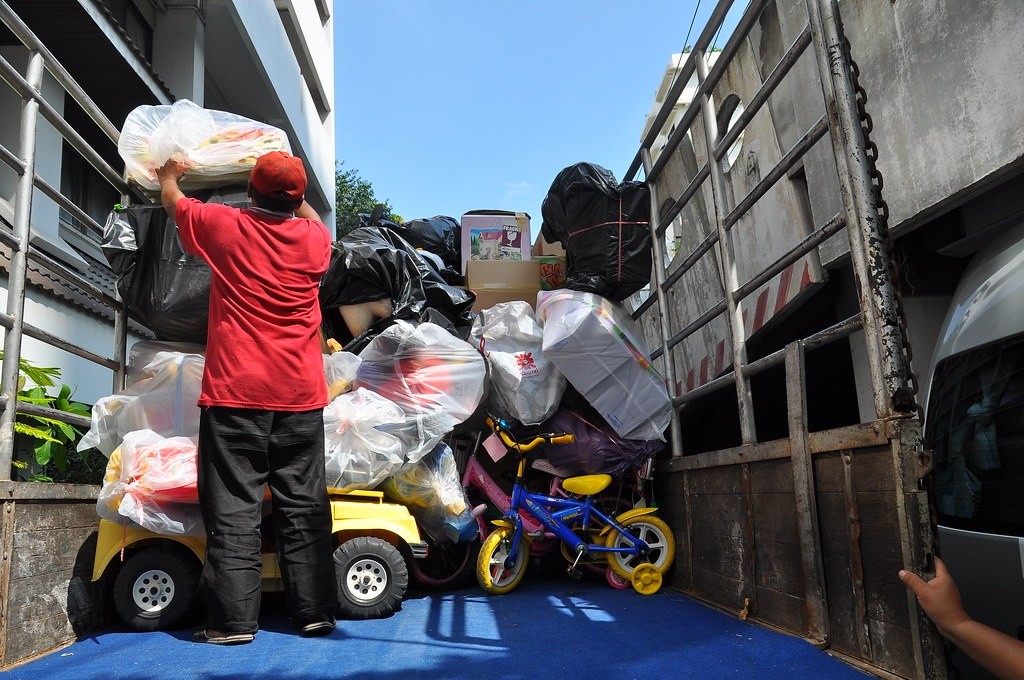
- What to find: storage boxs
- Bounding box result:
[532,256,565,291]
[461,214,531,276]
[531,230,566,256]
[465,259,541,315]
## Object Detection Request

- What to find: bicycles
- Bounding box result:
[399,407,650,591]
[476,406,677,598]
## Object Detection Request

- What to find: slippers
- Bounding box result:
[191,629,255,643]
[302,614,337,632]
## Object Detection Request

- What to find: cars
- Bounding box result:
[93,487,429,630]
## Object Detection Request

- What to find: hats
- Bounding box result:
[250,151,307,201]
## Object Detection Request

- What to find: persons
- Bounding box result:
[898,556,1024,680]
[155,151,337,644]
